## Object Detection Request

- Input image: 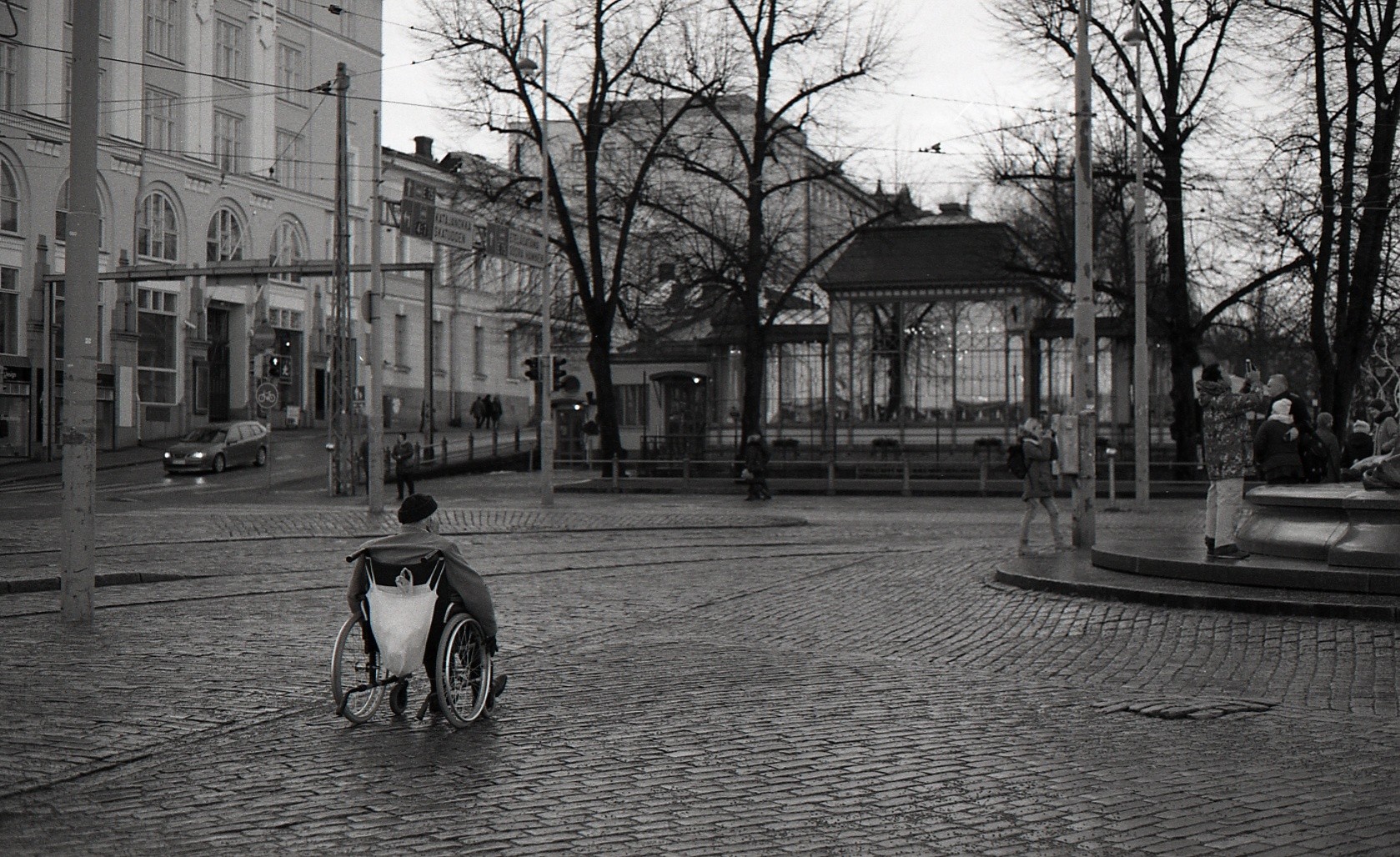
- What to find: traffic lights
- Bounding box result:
[523,354,541,381]
[549,355,570,392]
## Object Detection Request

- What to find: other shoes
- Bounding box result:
[474,675,507,708]
[1204,536,1215,556]
[1054,536,1071,550]
[1018,541,1037,556]
[1214,544,1250,559]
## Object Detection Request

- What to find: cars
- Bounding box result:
[162,419,265,474]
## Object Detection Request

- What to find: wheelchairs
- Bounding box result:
[329,546,507,732]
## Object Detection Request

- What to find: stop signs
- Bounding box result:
[253,324,275,349]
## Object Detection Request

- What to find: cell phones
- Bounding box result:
[1245,359,1252,374]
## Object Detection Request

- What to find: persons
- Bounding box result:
[1196,363,1263,559]
[1018,418,1080,555]
[348,494,508,707]
[743,428,771,501]
[361,435,368,495]
[393,431,415,499]
[1253,372,1397,484]
[469,393,502,429]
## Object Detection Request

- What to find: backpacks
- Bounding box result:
[1006,438,1033,480]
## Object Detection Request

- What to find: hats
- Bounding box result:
[397,494,438,525]
[400,432,406,440]
[1273,398,1292,414]
[1354,419,1368,426]
[1368,399,1385,411]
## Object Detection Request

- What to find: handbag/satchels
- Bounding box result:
[364,555,445,676]
[1300,436,1333,475]
